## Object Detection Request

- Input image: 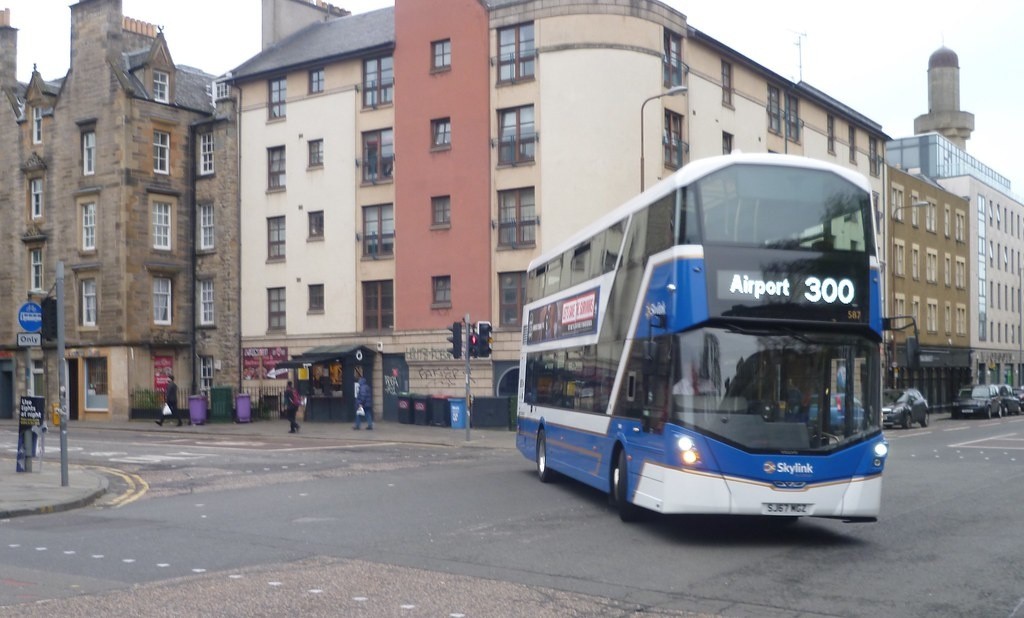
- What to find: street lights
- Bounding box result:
[639,86,691,192]
[894,199,929,388]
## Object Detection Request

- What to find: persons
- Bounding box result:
[672,352,716,395]
[284,381,302,434]
[155,375,183,427]
[352,378,374,431]
[785,378,804,423]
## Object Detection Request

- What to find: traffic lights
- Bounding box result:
[477,320,493,360]
[446,322,462,358]
[469,333,479,358]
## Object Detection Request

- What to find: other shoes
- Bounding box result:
[296,425,301,433]
[156,421,163,426]
[366,426,373,430]
[287,430,296,433]
[176,422,183,427]
[353,426,360,430]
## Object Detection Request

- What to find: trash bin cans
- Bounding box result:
[429,396,448,428]
[397,393,412,424]
[188,395,207,425]
[235,393,251,423]
[412,394,428,425]
[449,396,469,429]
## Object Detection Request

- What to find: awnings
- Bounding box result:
[275,356,338,370]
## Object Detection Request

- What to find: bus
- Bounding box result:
[514,148,921,526]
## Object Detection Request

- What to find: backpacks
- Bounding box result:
[287,388,301,407]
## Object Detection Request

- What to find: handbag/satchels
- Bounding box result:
[356,406,365,417]
[163,403,172,416]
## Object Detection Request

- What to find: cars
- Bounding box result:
[793,392,868,434]
[951,384,1024,419]
[866,387,931,429]
